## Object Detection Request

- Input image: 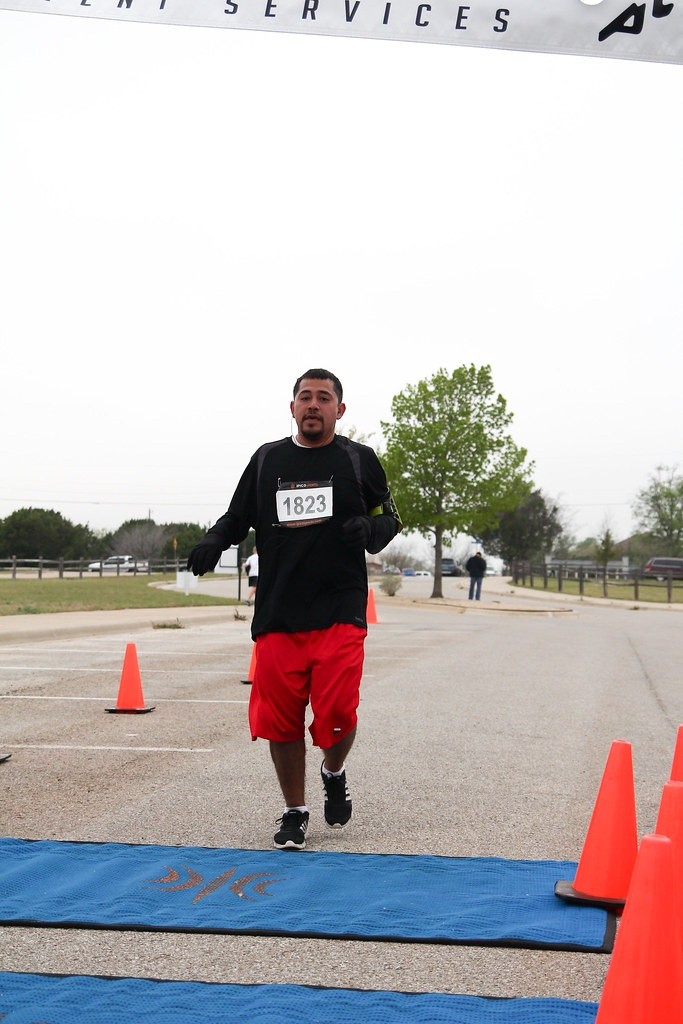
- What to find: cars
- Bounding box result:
[88,556,148,572]
[484,566,496,577]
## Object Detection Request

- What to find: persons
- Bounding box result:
[466,552,486,599]
[185,369,404,848]
[244,546,258,606]
[549,570,555,577]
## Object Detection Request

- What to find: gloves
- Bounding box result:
[341,516,371,552]
[186,535,228,576]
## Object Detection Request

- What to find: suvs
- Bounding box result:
[441,558,465,577]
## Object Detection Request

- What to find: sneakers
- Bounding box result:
[320,758,352,828]
[273,810,310,849]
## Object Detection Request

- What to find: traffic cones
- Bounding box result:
[554,739,639,910]
[366,587,381,624]
[104,643,156,713]
[595,725,683,1024]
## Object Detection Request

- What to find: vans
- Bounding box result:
[643,558,683,581]
